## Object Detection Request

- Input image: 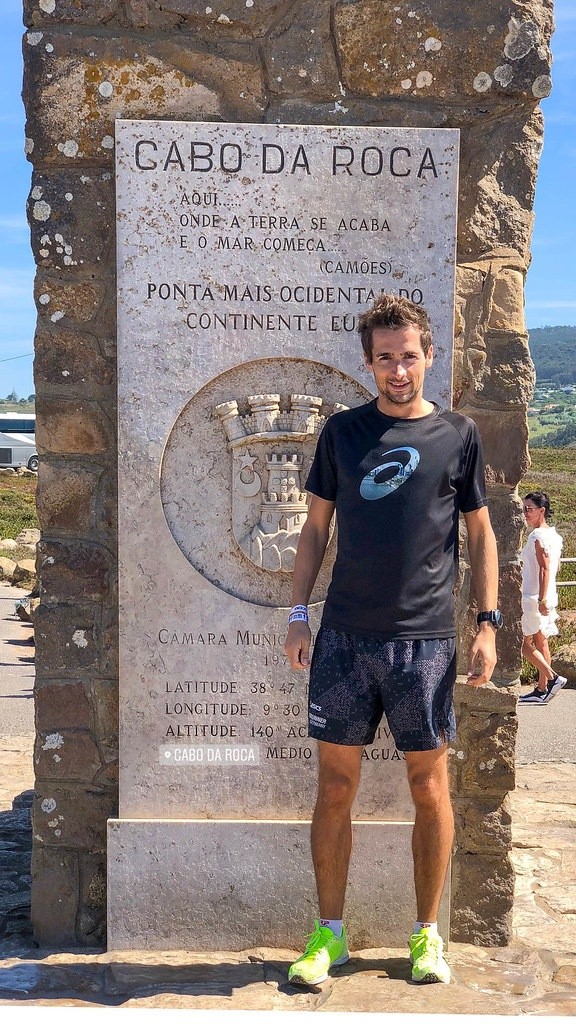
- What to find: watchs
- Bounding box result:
[537,598,548,605]
[477,609,504,630]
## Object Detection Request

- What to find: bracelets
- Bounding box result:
[287,605,308,625]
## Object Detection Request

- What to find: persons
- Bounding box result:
[518,492,568,705]
[283,294,504,989]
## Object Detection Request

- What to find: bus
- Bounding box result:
[0,412,39,471]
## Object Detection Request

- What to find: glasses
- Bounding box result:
[524,505,541,512]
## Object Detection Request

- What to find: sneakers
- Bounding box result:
[518,687,547,702]
[408,928,451,984]
[543,674,567,702]
[288,920,349,984]
